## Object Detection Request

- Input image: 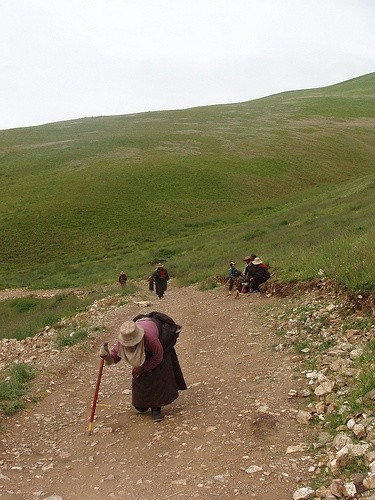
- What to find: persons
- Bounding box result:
[99,317,187,422]
[119,271,127,289]
[148,276,155,291]
[241,254,270,293]
[152,264,169,300]
[228,261,237,294]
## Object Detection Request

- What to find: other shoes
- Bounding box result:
[135,405,149,412]
[151,409,162,422]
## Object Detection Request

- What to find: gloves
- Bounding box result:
[98,345,114,365]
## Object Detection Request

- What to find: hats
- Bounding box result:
[252,257,263,265]
[120,271,124,275]
[156,263,163,267]
[118,320,144,347]
[243,256,252,261]
[229,262,236,267]
